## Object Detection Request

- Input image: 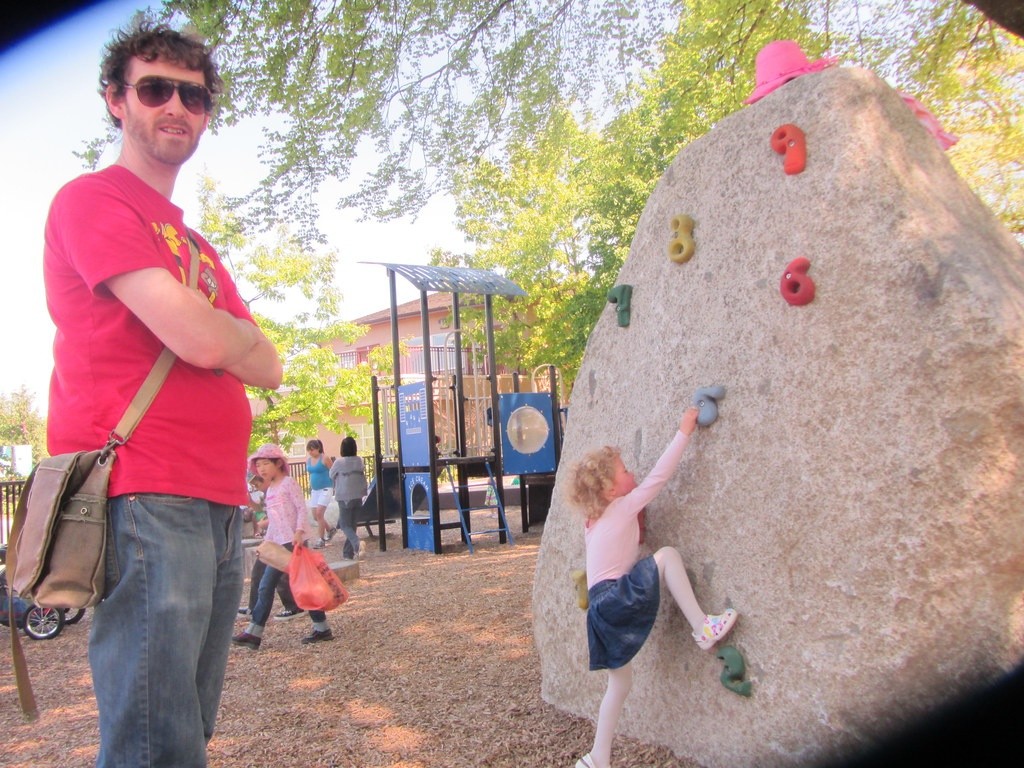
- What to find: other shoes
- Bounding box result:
[231,633,261,649]
[356,542,366,561]
[236,607,254,619]
[302,629,335,643]
[273,610,304,620]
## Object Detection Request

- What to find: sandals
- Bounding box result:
[575,753,597,767]
[693,609,738,650]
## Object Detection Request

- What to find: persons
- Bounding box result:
[329,436,368,561]
[564,408,739,768]
[45,24,284,768]
[305,441,336,550]
[230,442,335,651]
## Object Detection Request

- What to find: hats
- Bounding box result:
[744,40,837,104]
[247,444,290,477]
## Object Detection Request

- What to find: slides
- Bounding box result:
[336,463,447,530]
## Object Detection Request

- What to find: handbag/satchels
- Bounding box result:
[8,446,118,610]
[284,544,349,612]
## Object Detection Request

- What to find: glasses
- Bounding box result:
[123,75,213,116]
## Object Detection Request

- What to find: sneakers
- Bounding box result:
[313,539,325,549]
[325,528,337,541]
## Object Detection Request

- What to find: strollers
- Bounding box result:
[0,544,86,639]
